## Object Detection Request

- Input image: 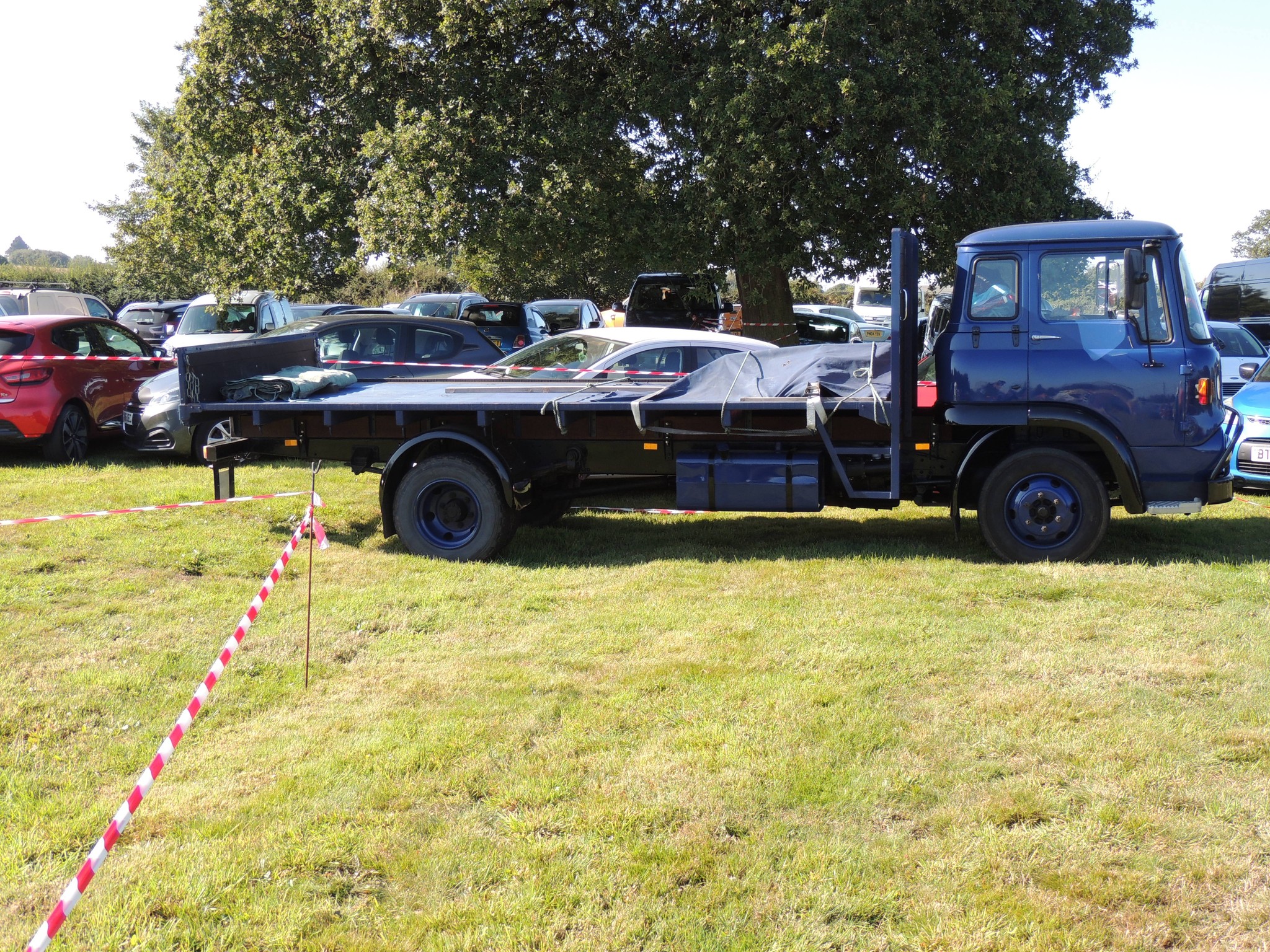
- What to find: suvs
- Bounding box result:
[0,281,118,341]
[0,314,178,463]
[918,291,953,363]
[397,293,500,324]
[116,299,193,348]
[721,304,743,337]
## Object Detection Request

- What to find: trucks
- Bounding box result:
[175,222,1242,563]
[846,271,925,329]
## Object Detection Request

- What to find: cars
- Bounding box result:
[1206,321,1270,493]
[792,305,891,343]
[529,298,607,337]
[600,287,684,328]
[458,301,561,357]
[118,314,511,468]
[289,302,366,322]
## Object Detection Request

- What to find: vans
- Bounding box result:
[612,272,735,333]
[161,290,294,358]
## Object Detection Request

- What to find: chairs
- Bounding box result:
[62,330,84,356]
[658,352,680,373]
[648,287,663,309]
[372,327,395,363]
[336,330,358,369]
[607,354,639,379]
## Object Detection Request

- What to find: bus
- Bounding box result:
[1197,258,1270,350]
